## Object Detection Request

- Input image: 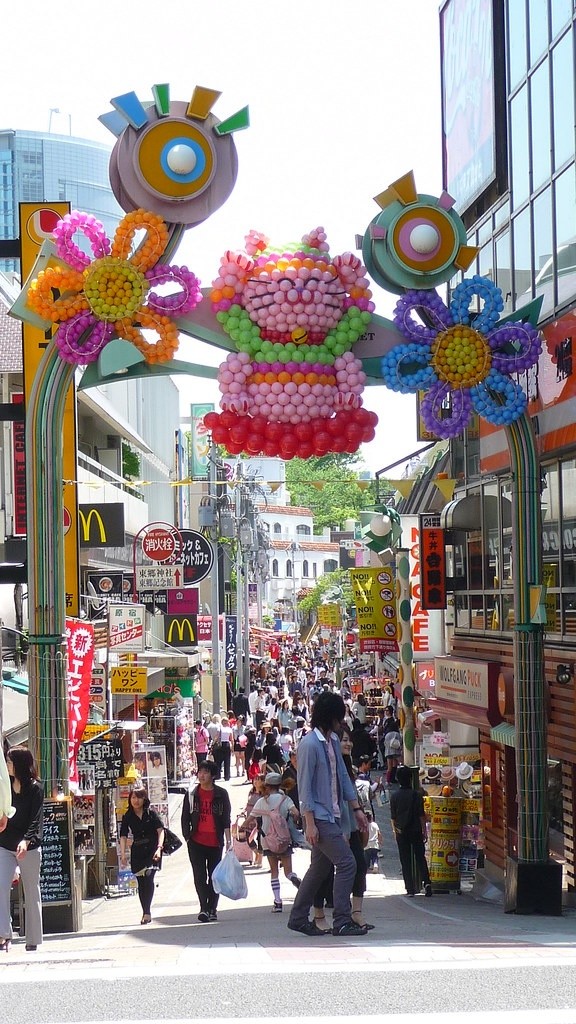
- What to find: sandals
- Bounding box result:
[351,911,375,930]
[312,915,333,934]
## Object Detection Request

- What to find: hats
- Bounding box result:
[265,772,282,785]
[418,760,482,796]
[262,722,271,727]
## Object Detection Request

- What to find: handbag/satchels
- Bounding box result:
[376,784,391,806]
[162,828,183,856]
[390,732,400,750]
[212,741,222,751]
[212,846,248,900]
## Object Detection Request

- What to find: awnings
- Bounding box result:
[489,721,516,747]
[417,709,441,723]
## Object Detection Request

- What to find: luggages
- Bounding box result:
[232,814,253,865]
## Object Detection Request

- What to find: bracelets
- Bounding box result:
[352,807,364,812]
[157,845,164,849]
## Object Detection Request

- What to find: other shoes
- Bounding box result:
[210,909,218,920]
[373,863,378,873]
[424,884,432,897]
[141,914,151,925]
[273,899,282,912]
[405,891,414,897]
[198,911,210,922]
[291,876,300,889]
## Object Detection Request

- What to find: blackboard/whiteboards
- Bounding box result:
[19,797,77,908]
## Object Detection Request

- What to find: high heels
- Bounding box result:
[0,937,12,952]
[26,945,37,951]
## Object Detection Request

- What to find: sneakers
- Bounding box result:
[287,919,324,936]
[333,921,368,935]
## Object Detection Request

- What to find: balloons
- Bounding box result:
[26,208,203,365]
[380,275,544,441]
[203,225,379,461]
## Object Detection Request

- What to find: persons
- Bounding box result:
[311,723,376,936]
[286,690,370,938]
[0,746,47,952]
[149,751,166,777]
[241,771,303,911]
[193,631,405,871]
[135,753,147,775]
[390,766,432,898]
[181,759,234,923]
[118,785,164,927]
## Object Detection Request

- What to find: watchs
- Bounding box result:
[226,840,232,844]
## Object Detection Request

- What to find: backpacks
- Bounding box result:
[251,795,290,854]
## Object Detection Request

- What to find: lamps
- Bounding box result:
[556,663,575,683]
